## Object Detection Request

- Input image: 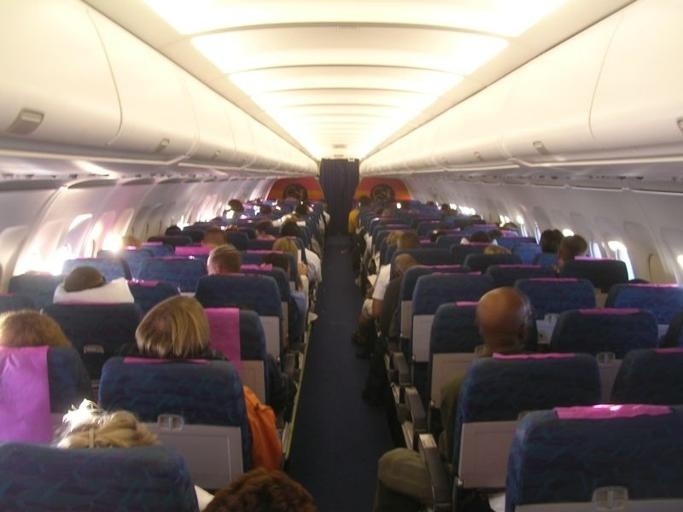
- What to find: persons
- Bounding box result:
[0,308,74,347]
[662,317,681,348]
[53,401,214,511]
[49,183,331,306]
[115,293,283,470]
[202,467,319,510]
[373,284,531,512]
[347,182,650,339]
